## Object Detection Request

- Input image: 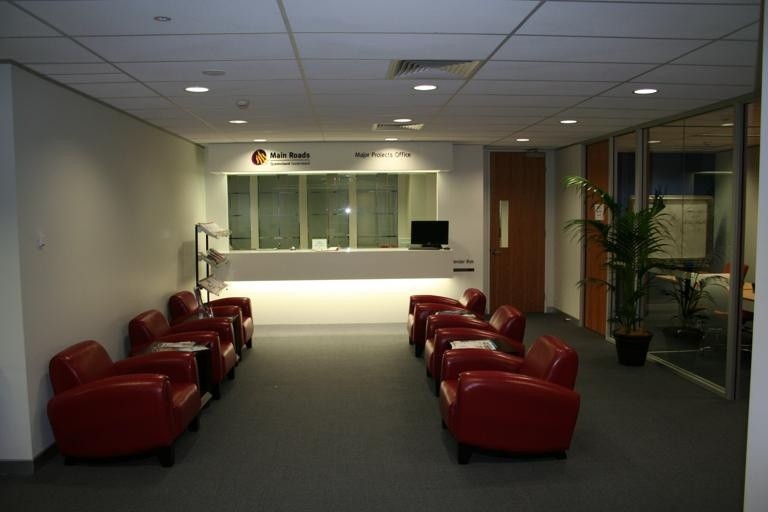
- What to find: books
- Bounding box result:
[207,248,226,265]
[198,221,226,237]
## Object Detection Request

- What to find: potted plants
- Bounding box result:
[562,175,665,367]
[665,254,709,347]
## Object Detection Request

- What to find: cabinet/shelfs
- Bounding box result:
[194,220,232,308]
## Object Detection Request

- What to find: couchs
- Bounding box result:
[126,309,238,401]
[167,290,254,361]
[404,285,487,360]
[437,333,582,466]
[46,340,202,469]
[424,302,527,397]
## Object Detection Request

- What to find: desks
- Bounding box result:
[432,308,475,317]
[141,342,217,417]
[655,270,756,359]
[446,339,517,357]
[179,315,242,366]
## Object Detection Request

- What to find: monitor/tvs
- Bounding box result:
[411,220,449,249]
